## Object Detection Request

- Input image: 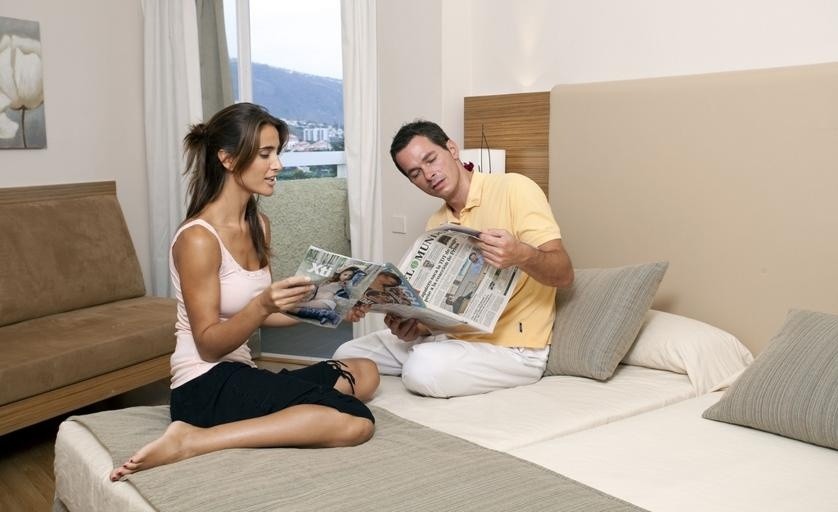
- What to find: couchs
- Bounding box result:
[0,179,182,436]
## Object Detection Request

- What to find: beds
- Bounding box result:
[51,57,835,510]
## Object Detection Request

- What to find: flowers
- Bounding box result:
[0,34,45,149]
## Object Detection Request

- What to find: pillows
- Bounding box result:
[622,308,756,387]
[544,262,671,382]
[702,311,838,451]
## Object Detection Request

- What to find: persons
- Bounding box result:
[445,292,472,313]
[368,271,402,294]
[292,265,360,326]
[468,252,485,272]
[108,103,380,482]
[328,119,576,400]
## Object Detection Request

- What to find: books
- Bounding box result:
[277,245,428,330]
[364,223,520,335]
